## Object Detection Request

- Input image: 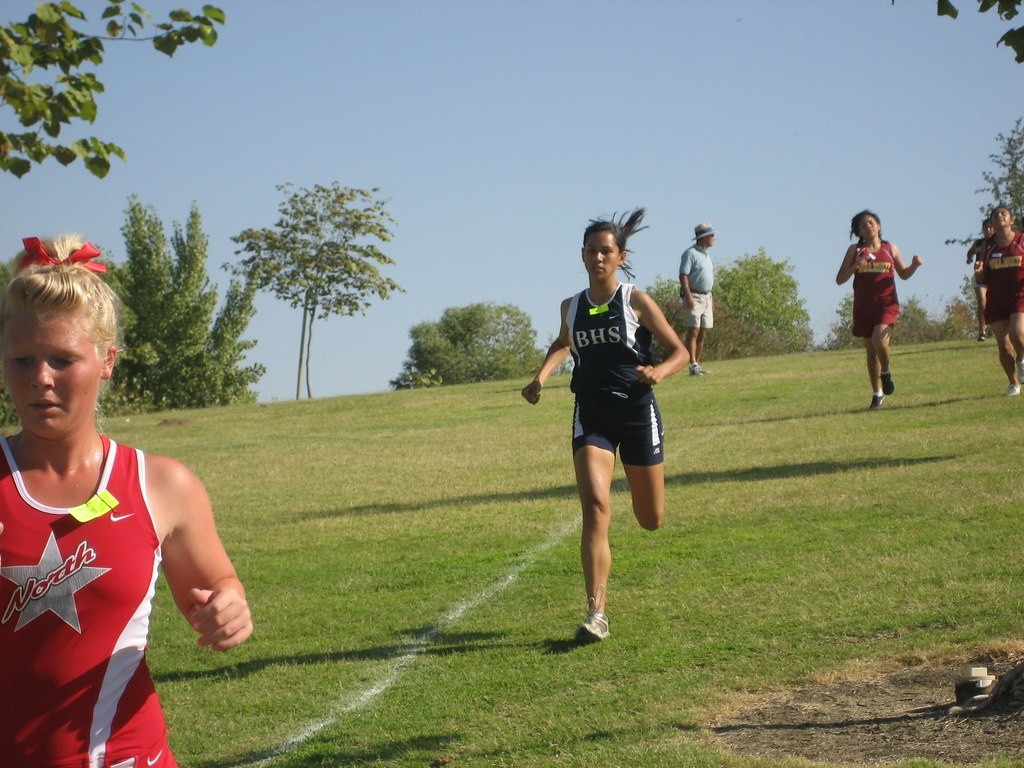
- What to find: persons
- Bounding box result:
[835,209,924,411]
[678,222,716,377]
[522,205,690,643]
[0,233,253,768]
[966,206,1024,397]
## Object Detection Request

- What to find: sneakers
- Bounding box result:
[689,365,703,375]
[1007,383,1021,396]
[574,584,610,641]
[698,365,710,374]
[1016,361,1024,384]
[978,332,986,341]
[870,393,885,409]
[880,370,894,395]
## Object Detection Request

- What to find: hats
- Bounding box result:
[691,222,717,241]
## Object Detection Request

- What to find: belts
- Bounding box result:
[690,289,710,295]
